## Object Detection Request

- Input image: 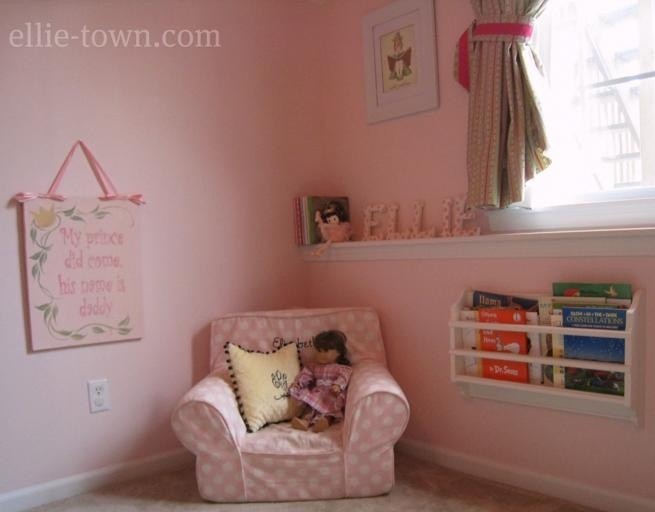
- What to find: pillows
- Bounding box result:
[222,339,305,434]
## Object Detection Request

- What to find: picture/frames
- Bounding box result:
[361,0,441,125]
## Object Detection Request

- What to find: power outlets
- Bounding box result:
[86,378,109,413]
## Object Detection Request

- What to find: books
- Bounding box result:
[293,196,347,244]
[460,282,632,396]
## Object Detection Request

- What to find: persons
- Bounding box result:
[310,202,353,258]
[289,329,352,433]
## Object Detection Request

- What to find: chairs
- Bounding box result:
[169,304,410,503]
